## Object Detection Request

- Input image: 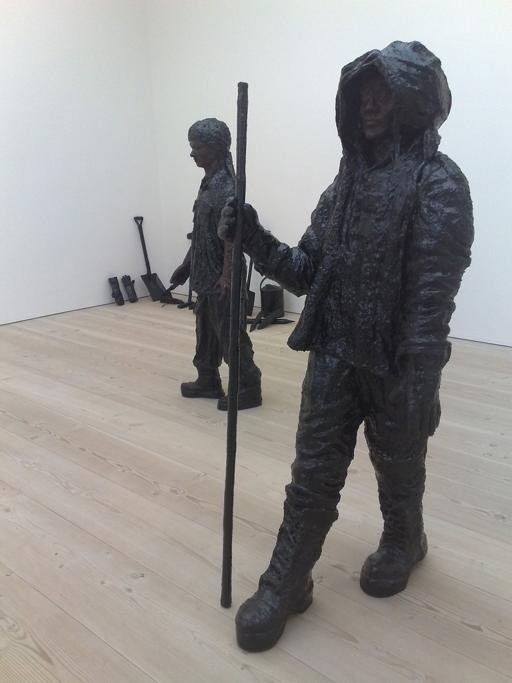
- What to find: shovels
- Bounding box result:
[246,257,256,317]
[134,217,167,301]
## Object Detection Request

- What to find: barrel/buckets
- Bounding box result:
[258,275,285,319]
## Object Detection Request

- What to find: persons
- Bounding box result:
[217,38,478,654]
[170,116,263,410]
